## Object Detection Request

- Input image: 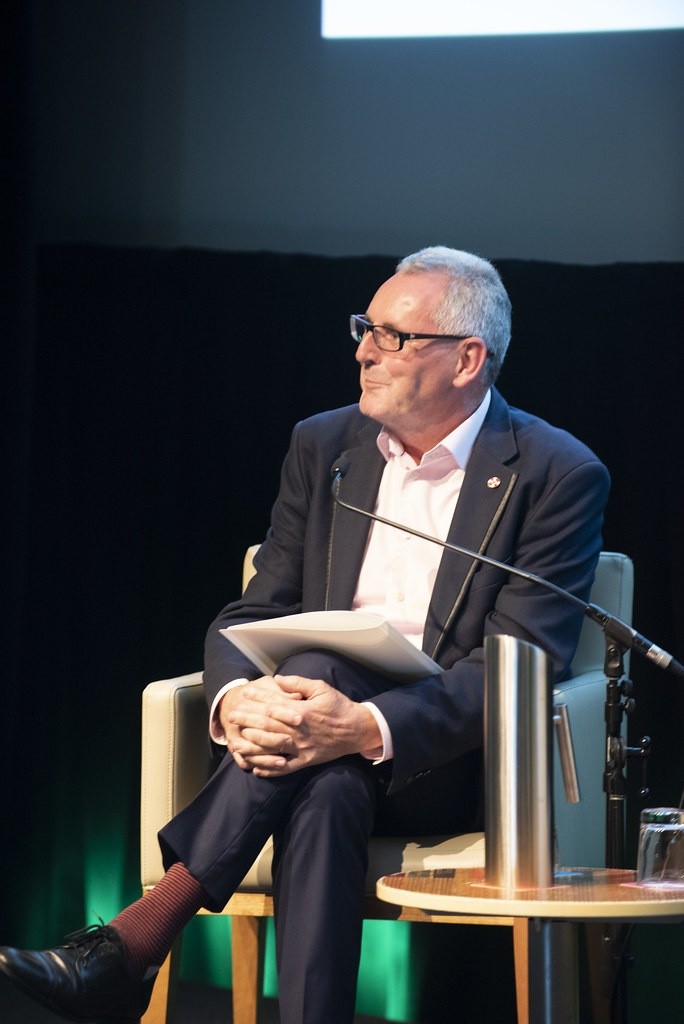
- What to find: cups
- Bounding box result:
[636,806,684,890]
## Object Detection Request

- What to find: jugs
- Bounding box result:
[483,635,579,891]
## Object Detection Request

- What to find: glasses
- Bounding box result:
[349,314,496,360]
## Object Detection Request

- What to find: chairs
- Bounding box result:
[140,542,636,1024]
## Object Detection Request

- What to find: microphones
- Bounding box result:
[330,456,676,670]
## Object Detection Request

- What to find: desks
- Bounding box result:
[375,867,684,1024]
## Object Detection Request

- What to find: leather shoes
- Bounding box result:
[0,917,159,1024]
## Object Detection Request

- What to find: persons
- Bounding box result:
[0,242,610,1024]
